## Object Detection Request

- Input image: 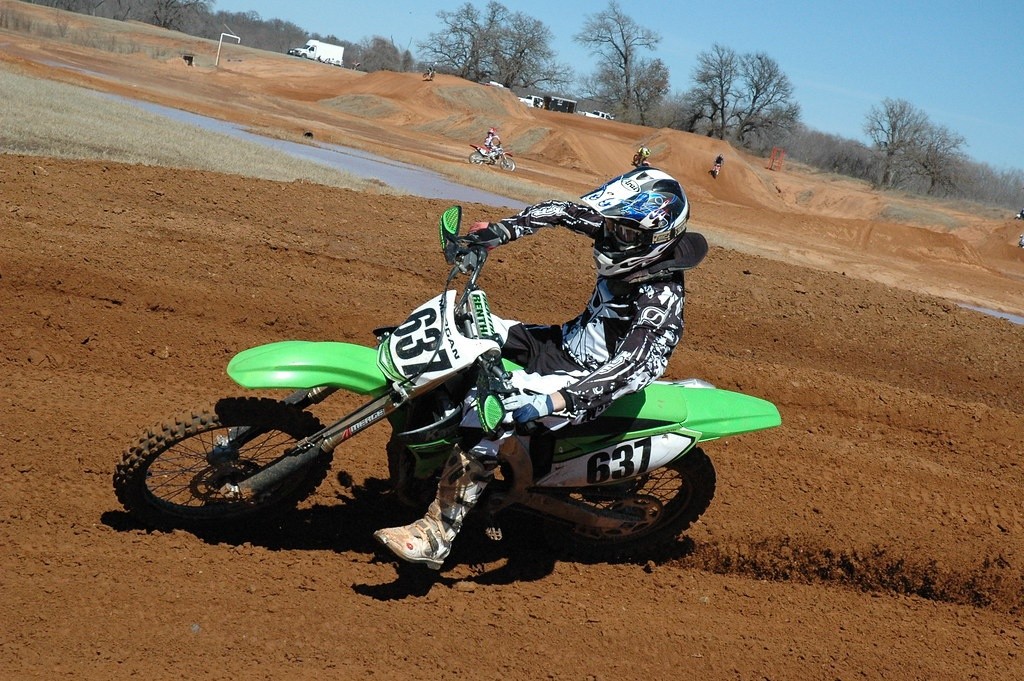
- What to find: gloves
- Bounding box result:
[503,394,554,424]
[458,221,503,250]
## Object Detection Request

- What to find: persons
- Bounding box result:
[429,64,436,79]
[637,143,651,166]
[712,153,725,171]
[484,127,502,163]
[374,164,691,573]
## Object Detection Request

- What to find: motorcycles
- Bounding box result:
[468,142,517,173]
[712,163,722,176]
[633,154,651,170]
[111,206,784,561]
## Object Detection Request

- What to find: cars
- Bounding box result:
[288,48,300,57]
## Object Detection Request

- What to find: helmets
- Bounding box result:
[487,127,497,136]
[580,167,690,276]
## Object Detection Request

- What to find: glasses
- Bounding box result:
[603,218,647,247]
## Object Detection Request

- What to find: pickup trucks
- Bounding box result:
[517,94,544,108]
[578,109,616,121]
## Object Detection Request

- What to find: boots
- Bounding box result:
[374,443,498,569]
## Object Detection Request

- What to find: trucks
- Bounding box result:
[294,39,344,67]
